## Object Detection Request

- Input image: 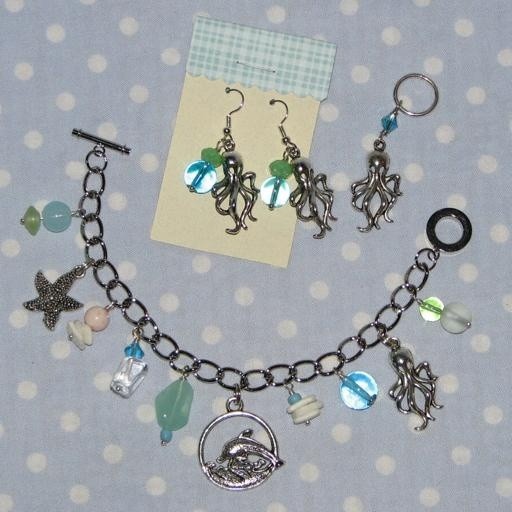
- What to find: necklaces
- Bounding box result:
[21,129,472,491]
